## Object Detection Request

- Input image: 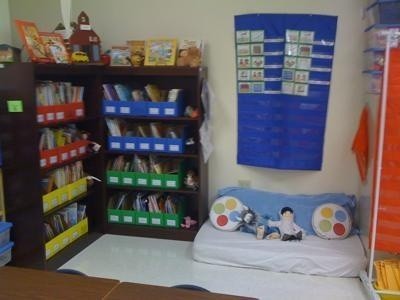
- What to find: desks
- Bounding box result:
[0,267,260,300]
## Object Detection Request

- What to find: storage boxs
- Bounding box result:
[361,0,400,95]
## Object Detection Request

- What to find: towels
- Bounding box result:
[351,102,368,183]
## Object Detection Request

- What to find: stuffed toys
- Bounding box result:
[267,207,306,241]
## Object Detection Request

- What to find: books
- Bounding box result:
[101,83,184,102]
[42,202,87,241]
[107,189,179,213]
[35,80,85,107]
[39,160,84,194]
[106,152,180,174]
[38,126,82,152]
[104,115,186,139]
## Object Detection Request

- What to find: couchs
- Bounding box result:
[192,188,368,278]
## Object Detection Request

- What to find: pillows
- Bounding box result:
[209,195,250,231]
[310,202,352,239]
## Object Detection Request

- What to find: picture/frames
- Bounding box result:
[111,38,177,66]
[15,20,72,63]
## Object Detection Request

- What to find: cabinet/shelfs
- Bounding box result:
[0,62,96,264]
[95,64,209,241]
